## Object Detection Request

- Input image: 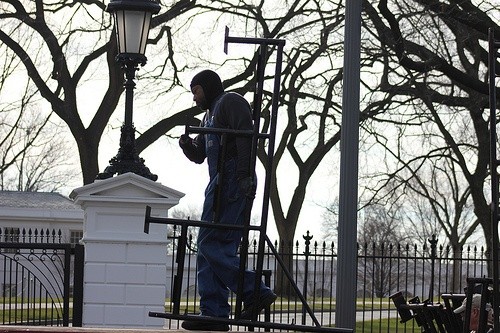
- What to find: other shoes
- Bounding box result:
[182,322,230,332]
[240,293,278,320]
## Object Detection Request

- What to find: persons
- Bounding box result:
[453,294,494,333]
[179,69,277,331]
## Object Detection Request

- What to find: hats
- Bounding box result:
[453,293,481,314]
[191,70,224,103]
